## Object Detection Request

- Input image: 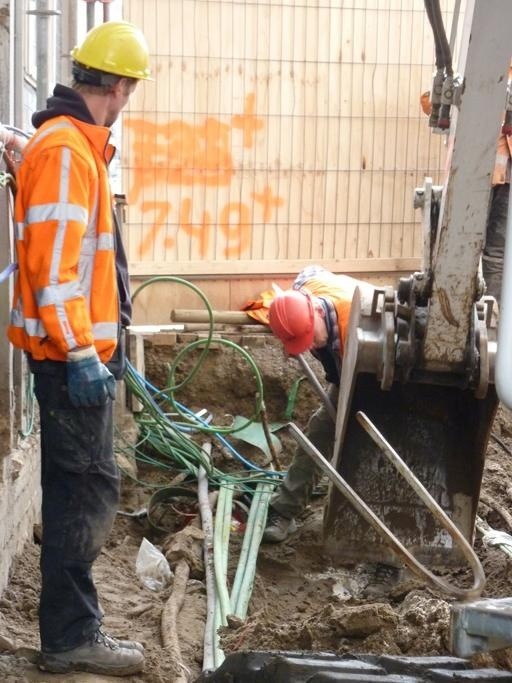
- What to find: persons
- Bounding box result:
[261,265,395,543]
[5,20,155,675]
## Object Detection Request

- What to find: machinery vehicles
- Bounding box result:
[323,0,512,568]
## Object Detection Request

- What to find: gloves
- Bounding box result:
[66,345,116,408]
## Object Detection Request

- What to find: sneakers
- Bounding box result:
[264,514,298,543]
[363,562,405,598]
[37,630,145,676]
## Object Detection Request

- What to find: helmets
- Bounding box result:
[269,290,314,355]
[70,21,155,81]
